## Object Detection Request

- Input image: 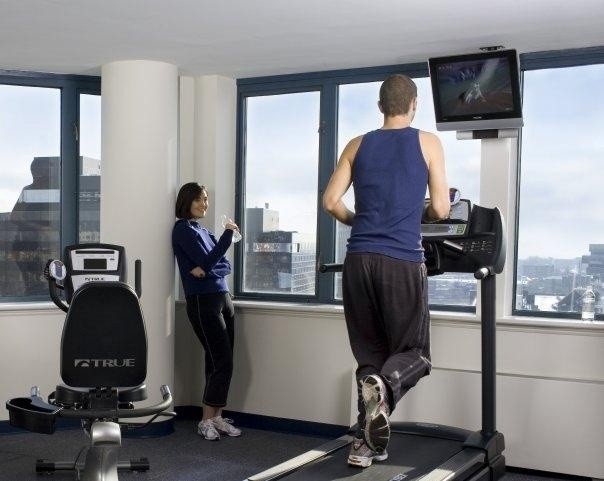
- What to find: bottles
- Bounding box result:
[220,216,242,244]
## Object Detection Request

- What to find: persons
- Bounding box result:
[321,73,458,468]
[171,183,243,441]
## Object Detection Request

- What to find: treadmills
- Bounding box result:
[244,187,506,481]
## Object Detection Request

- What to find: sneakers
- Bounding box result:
[346,437,389,469]
[197,419,220,440]
[361,372,391,454]
[213,418,242,437]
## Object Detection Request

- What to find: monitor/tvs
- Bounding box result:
[427,48,525,132]
